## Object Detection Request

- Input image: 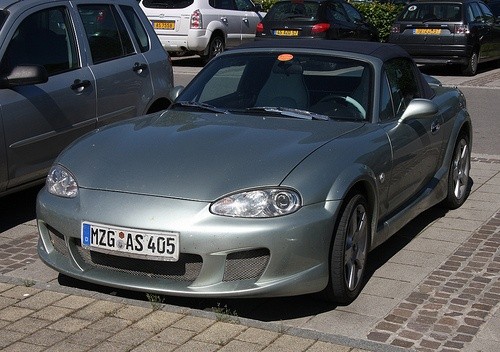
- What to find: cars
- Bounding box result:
[254,0,380,70]
[57,8,113,40]
[389,1,500,76]
[0,0,188,201]
[141,0,268,63]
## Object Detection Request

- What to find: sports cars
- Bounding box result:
[35,38,473,307]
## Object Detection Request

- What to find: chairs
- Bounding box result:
[254,58,310,110]
[347,66,396,120]
